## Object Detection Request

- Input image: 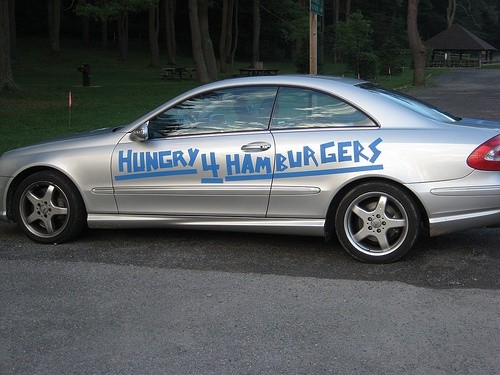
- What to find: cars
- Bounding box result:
[0,74,500,264]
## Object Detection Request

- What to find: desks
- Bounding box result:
[239,69,277,74]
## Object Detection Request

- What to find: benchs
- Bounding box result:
[234,74,253,77]
[159,65,197,80]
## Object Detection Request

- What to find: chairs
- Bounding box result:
[253,98,279,129]
[233,97,253,124]
